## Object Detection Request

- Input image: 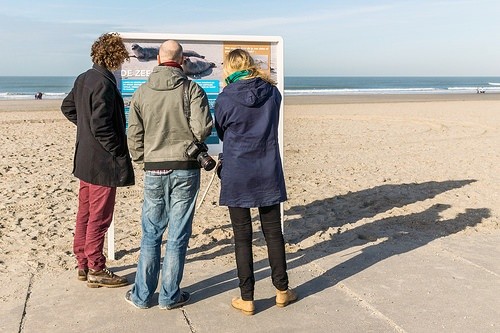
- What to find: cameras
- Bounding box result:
[186,141,216,171]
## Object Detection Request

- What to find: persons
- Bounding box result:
[124,40,214,309]
[213,48,297,314]
[61,33,135,288]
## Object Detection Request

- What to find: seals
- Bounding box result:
[131,44,205,62]
[182,56,217,80]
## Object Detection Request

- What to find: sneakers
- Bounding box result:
[124,289,151,309]
[276,286,299,306]
[231,295,256,315]
[78,268,129,288]
[158,291,190,310]
